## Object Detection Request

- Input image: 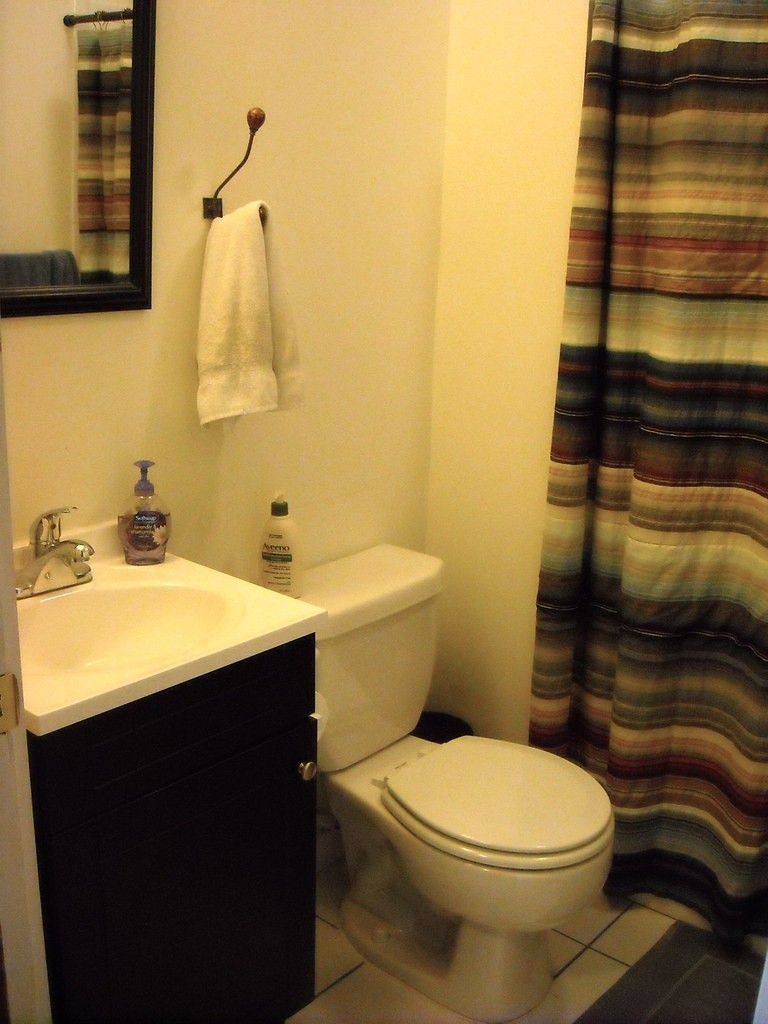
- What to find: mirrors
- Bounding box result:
[0,0,156,319]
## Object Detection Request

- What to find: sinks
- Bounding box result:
[12,548,329,737]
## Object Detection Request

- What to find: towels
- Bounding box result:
[199,201,304,426]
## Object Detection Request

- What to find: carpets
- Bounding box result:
[572,919,765,1024]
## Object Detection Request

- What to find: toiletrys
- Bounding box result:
[118,461,170,565]
[260,490,298,596]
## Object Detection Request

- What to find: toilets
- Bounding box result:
[261,542,615,1024]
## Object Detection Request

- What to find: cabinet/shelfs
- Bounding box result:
[27,612,318,1024]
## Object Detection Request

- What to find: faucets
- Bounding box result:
[28,505,93,563]
